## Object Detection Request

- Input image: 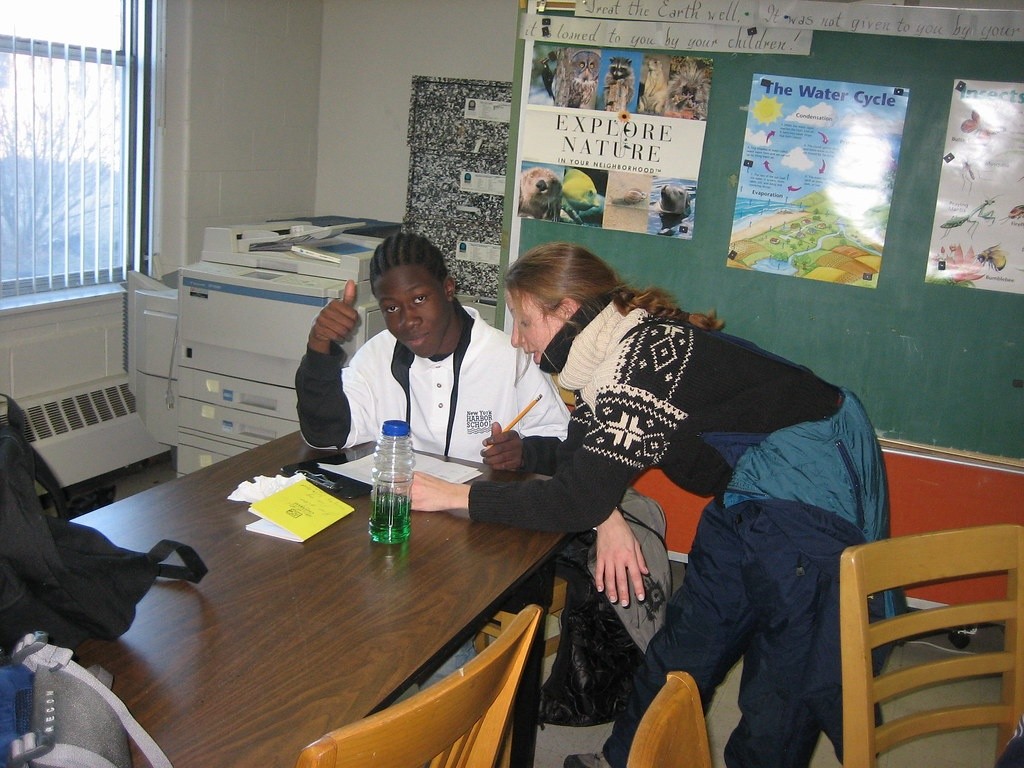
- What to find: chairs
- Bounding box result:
[625,671,712,768]
[475,576,566,663]
[293,602,545,768]
[839,524,1024,768]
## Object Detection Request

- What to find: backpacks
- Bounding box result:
[0,394,208,635]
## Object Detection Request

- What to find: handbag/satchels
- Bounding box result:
[6,633,174,768]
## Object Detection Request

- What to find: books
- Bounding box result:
[245,479,355,543]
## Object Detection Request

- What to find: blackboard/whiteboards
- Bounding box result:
[486,2,1024,478]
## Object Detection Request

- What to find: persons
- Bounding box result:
[295,233,571,462]
[412,243,890,768]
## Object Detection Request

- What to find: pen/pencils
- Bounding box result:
[483,394,543,455]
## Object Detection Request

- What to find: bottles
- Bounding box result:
[367,421,416,545]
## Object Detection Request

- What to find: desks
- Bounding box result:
[70,434,579,768]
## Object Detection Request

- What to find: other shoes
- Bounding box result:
[563,753,612,768]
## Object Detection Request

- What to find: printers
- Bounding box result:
[129,221,420,480]
